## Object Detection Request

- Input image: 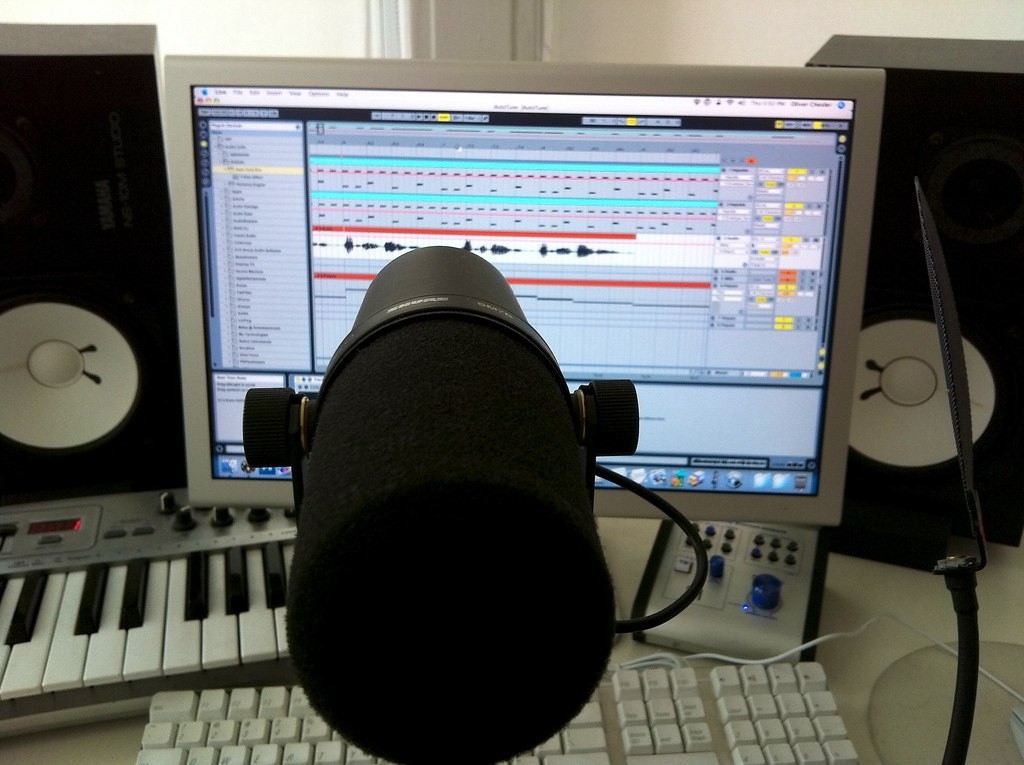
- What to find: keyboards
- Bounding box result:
[133,657,860,764]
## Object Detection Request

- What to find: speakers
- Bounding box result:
[0,22,190,502]
[799,35,1024,545]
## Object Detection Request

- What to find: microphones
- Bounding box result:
[284,245,615,764]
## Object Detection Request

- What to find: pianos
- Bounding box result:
[0,483,300,738]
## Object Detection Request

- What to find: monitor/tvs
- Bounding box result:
[151,57,887,535]
